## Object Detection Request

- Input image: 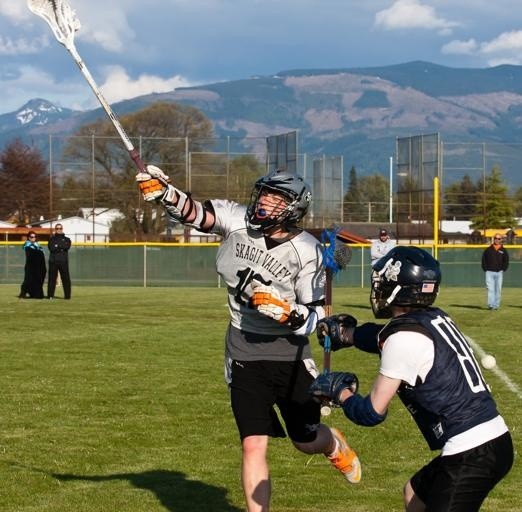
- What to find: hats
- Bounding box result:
[380,229,387,236]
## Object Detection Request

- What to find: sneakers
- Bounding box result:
[326,427,362,483]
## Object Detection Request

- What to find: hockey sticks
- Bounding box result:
[29,0,147,174]
[317,223,351,417]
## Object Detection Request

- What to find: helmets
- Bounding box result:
[247,168,311,231]
[369,245,441,318]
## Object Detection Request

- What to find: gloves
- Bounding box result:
[309,372,359,407]
[136,164,172,201]
[251,279,294,322]
[316,314,357,351]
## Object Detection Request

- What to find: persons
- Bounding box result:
[48,223,72,299]
[310,246,513,512]
[135,164,361,511]
[19,230,46,298]
[371,228,395,271]
[481,233,508,308]
